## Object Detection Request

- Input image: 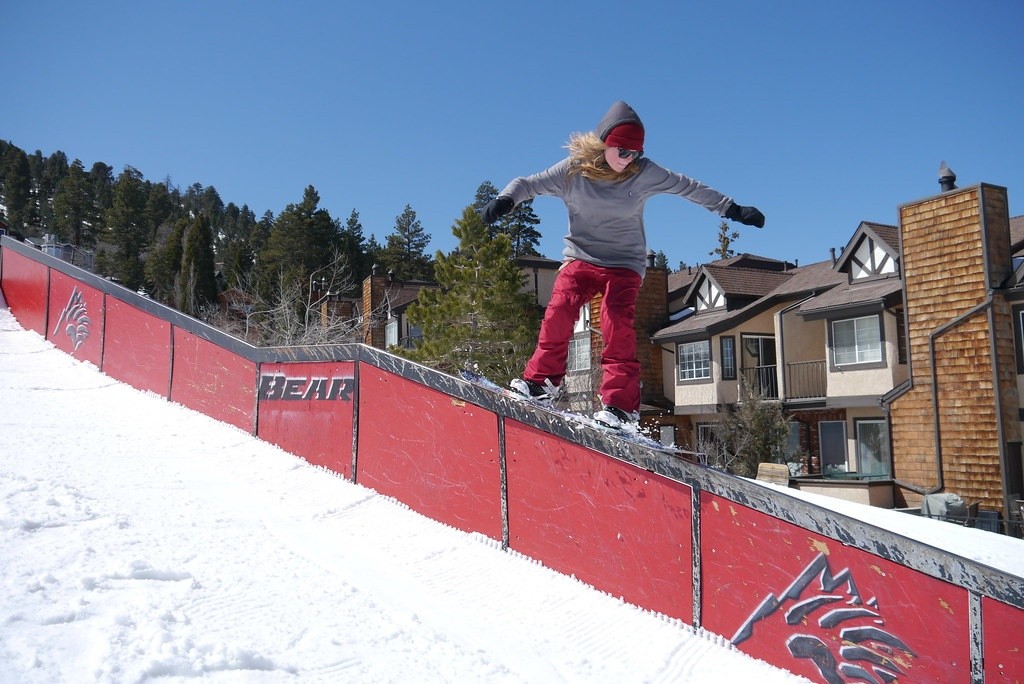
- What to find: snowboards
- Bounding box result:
[456,366,706,457]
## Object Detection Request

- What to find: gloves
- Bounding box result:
[721,203,765,228]
[482,196,514,225]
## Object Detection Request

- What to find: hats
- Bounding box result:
[603,123,644,151]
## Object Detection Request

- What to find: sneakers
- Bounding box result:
[595,405,640,426]
[510,378,559,403]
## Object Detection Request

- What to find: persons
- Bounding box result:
[480,99,766,427]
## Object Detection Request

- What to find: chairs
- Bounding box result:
[948,501,982,527]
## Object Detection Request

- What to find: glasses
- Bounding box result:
[613,146,644,160]
